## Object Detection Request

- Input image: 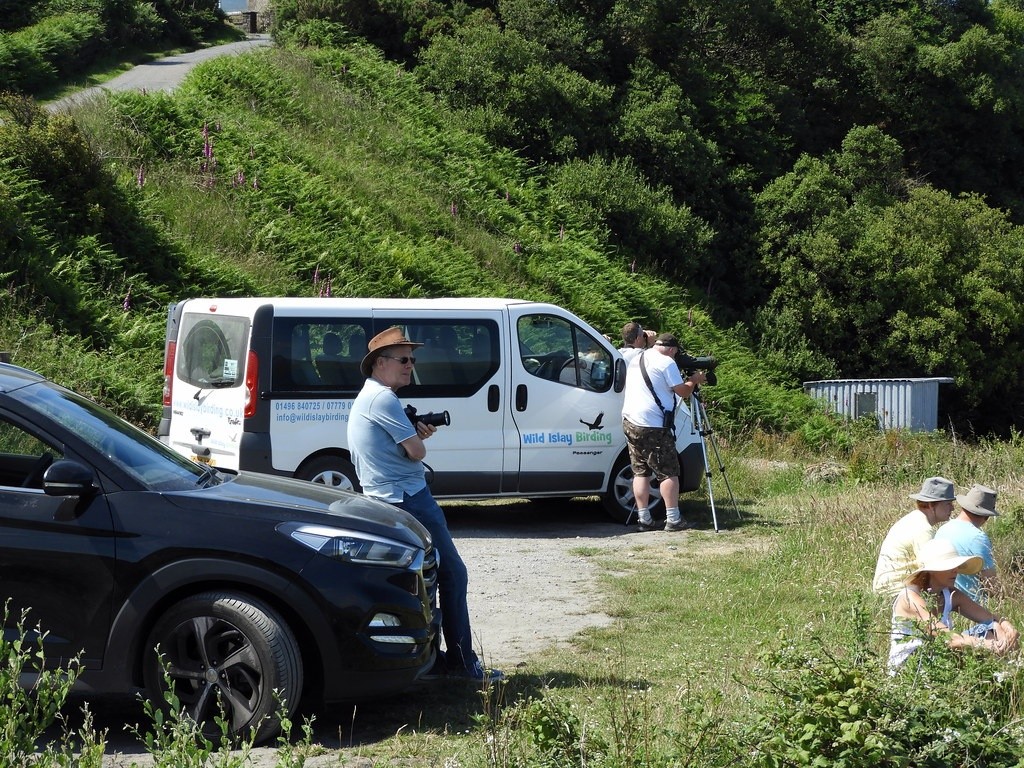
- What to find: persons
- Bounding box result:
[934,483,1005,606]
[622,333,708,532]
[618,322,655,363]
[888,539,1020,678]
[873,477,956,598]
[560,358,601,390]
[347,327,506,683]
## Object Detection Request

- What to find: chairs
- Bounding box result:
[338,335,367,390]
[431,327,462,386]
[316,332,342,385]
[463,333,493,387]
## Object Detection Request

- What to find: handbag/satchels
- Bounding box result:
[663,410,677,441]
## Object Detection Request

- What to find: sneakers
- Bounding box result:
[663,520,697,532]
[636,520,665,531]
[443,658,505,683]
[420,669,437,680]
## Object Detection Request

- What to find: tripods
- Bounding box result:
[625,370,743,532]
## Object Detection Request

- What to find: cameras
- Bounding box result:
[404,404,450,431]
[676,352,717,373]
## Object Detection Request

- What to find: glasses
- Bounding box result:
[378,354,416,365]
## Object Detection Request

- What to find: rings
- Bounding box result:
[424,433,427,435]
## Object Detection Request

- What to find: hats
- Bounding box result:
[902,538,983,588]
[360,326,424,378]
[908,478,956,503]
[654,333,684,351]
[957,484,1000,517]
